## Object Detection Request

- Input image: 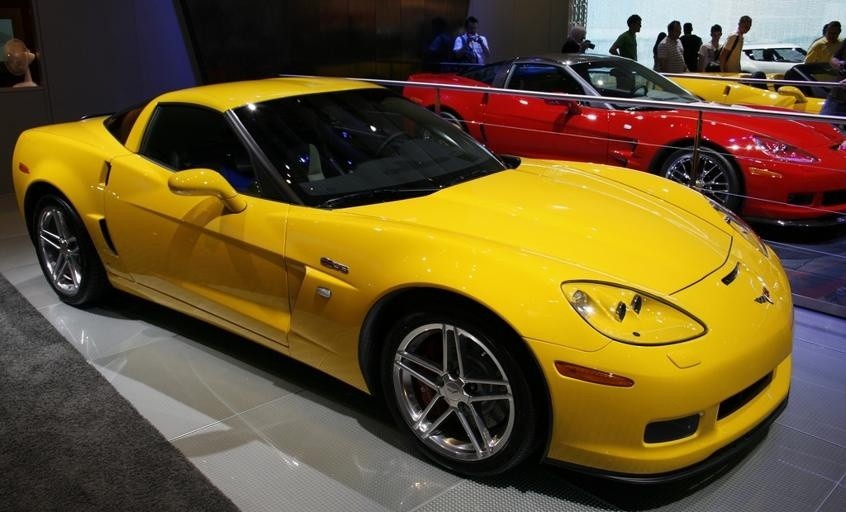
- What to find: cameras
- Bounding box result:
[585,40,594,49]
[469,33,479,42]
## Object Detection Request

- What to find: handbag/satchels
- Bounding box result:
[459,46,478,71]
[705,60,723,71]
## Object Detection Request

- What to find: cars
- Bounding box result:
[733,44,818,74]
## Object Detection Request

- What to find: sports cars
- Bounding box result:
[596,69,828,115]
[7,76,799,491]
[399,51,846,232]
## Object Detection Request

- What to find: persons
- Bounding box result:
[453,15,491,64]
[560,15,846,89]
